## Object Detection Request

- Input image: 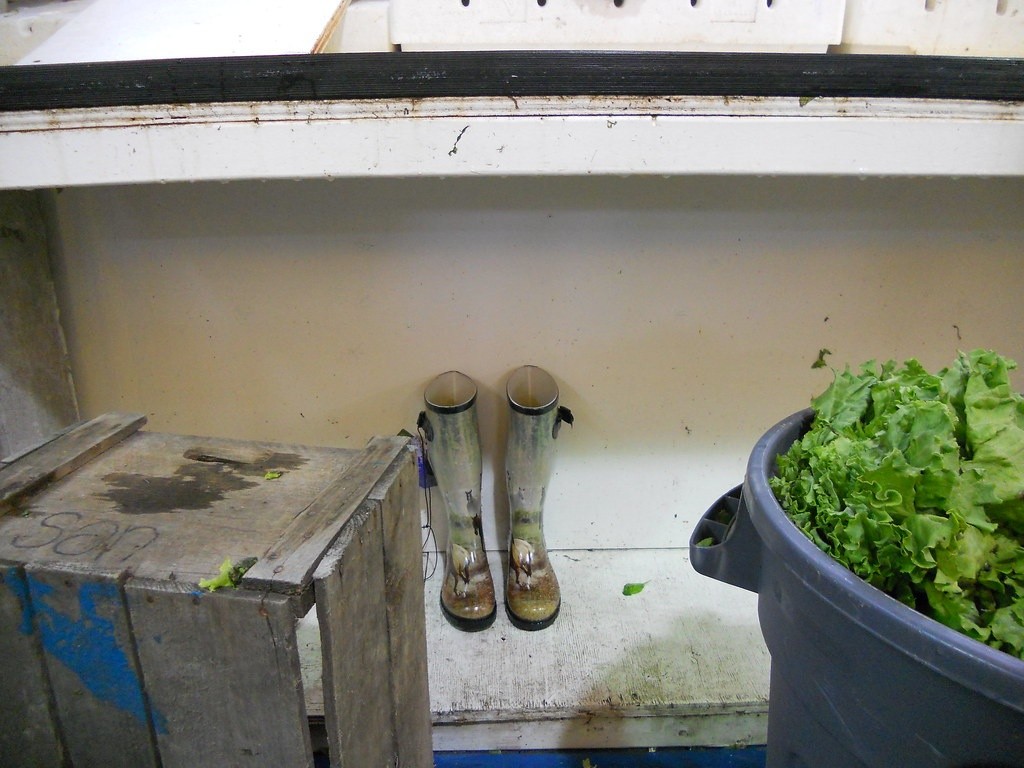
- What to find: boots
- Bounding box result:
[418,371,497,632]
[505,365,574,631]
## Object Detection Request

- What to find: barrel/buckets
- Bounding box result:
[690,401,1024,768]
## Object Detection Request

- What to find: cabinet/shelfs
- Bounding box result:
[0,97,1024,751]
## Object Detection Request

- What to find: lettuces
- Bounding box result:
[768,347,1023,671]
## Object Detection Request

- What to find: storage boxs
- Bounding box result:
[0,413,433,768]
